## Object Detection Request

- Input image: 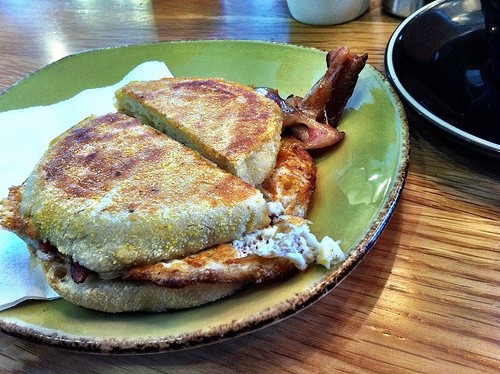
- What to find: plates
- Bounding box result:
[383,0,500,178]
[1,38,413,357]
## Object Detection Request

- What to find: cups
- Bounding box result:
[381,0,434,20]
[286,0,369,27]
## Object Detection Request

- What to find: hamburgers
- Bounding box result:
[0,76,317,311]
